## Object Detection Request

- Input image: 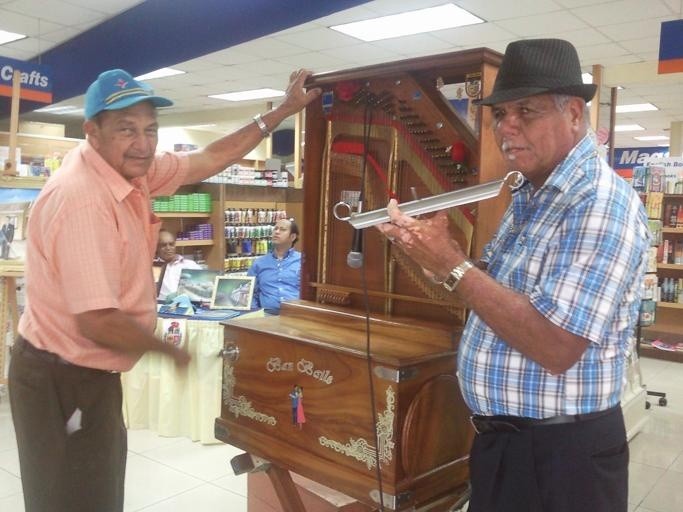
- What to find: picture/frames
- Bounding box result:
[0,201,30,269]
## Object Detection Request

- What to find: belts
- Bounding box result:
[469,412,606,437]
[17,335,71,366]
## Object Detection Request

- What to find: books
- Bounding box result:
[627,165,683,356]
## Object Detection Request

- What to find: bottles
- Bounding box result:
[223,206,286,225]
[663,202,683,229]
[659,275,683,304]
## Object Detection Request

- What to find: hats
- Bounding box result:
[472,40,597,106]
[85,69,173,120]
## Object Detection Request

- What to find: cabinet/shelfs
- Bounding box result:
[0,132,291,274]
[215,44,526,511]
[637,189,683,363]
[0,271,29,387]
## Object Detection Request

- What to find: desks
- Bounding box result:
[122,308,267,447]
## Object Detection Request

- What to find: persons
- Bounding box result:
[149,228,201,302]
[246,218,302,313]
[374,40,652,512]
[0,216,15,262]
[6,66,323,511]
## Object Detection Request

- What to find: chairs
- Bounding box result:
[636,298,667,409]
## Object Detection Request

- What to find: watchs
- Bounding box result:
[440,259,474,291]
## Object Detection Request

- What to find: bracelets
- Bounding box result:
[253,114,268,135]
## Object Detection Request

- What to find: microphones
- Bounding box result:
[346,200,364,269]
